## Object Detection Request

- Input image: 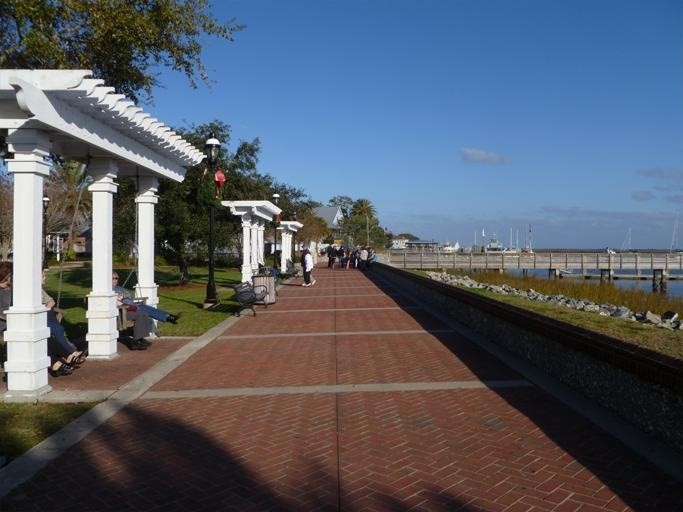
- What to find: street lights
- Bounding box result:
[293,211,296,257]
[204,133,222,307]
[43,193,50,268]
[272,193,280,274]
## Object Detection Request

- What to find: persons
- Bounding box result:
[0,261,86,377]
[327,243,377,271]
[112,270,182,350]
[301,245,316,287]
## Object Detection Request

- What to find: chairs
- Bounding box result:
[84,291,149,342]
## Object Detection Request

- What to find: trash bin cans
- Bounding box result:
[294,263,303,277]
[252,268,275,305]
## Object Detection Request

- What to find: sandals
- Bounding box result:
[52,349,88,377]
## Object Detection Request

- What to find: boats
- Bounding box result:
[482,225,534,253]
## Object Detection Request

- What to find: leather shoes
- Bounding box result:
[130,339,152,350]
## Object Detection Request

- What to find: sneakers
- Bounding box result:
[302,279,316,287]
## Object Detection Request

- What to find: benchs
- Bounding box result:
[232,280,269,317]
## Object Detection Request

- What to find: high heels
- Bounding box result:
[167,312,183,324]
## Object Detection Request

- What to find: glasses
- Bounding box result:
[111,277,119,280]
